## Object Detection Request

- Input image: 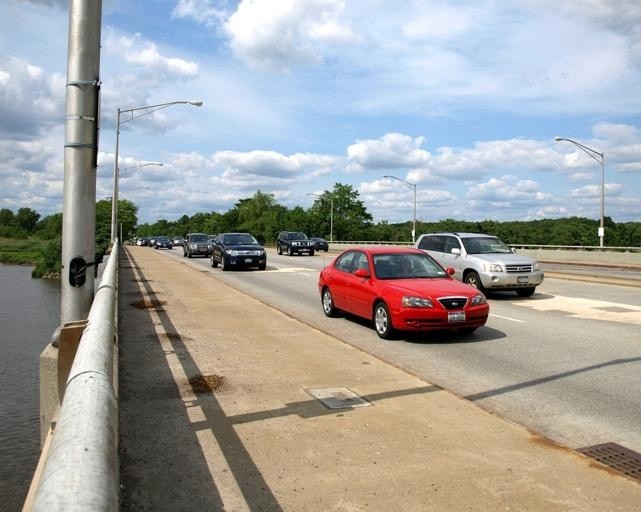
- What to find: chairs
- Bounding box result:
[376,259,391,275]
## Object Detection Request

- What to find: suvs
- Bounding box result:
[415,232,544,297]
[276,231,316,256]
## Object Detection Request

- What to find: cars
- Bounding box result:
[318,245,489,340]
[129,236,187,249]
[183,233,266,271]
[308,237,328,252]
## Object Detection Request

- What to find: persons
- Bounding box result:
[395,259,417,277]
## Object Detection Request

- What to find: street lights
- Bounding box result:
[110,101,203,244]
[382,175,416,242]
[554,137,604,252]
[305,193,334,241]
